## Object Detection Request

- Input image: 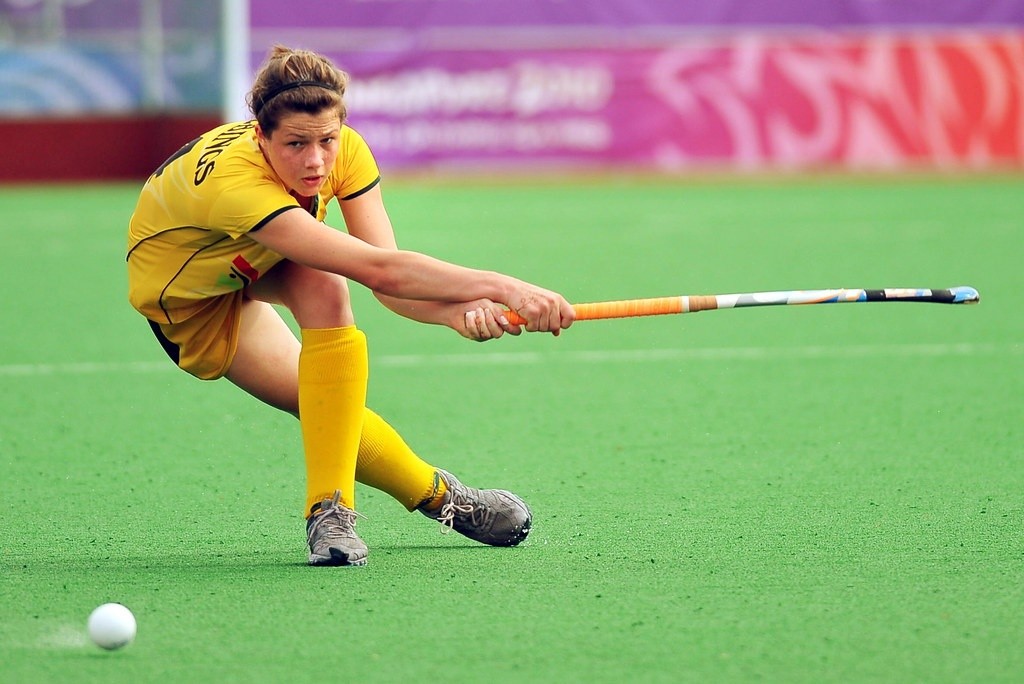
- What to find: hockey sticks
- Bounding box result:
[459,281,985,334]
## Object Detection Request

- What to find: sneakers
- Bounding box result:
[419,467,533,544]
[303,491,369,567]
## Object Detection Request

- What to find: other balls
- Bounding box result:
[84,600,140,654]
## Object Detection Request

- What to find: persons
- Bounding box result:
[127,45,574,566]
